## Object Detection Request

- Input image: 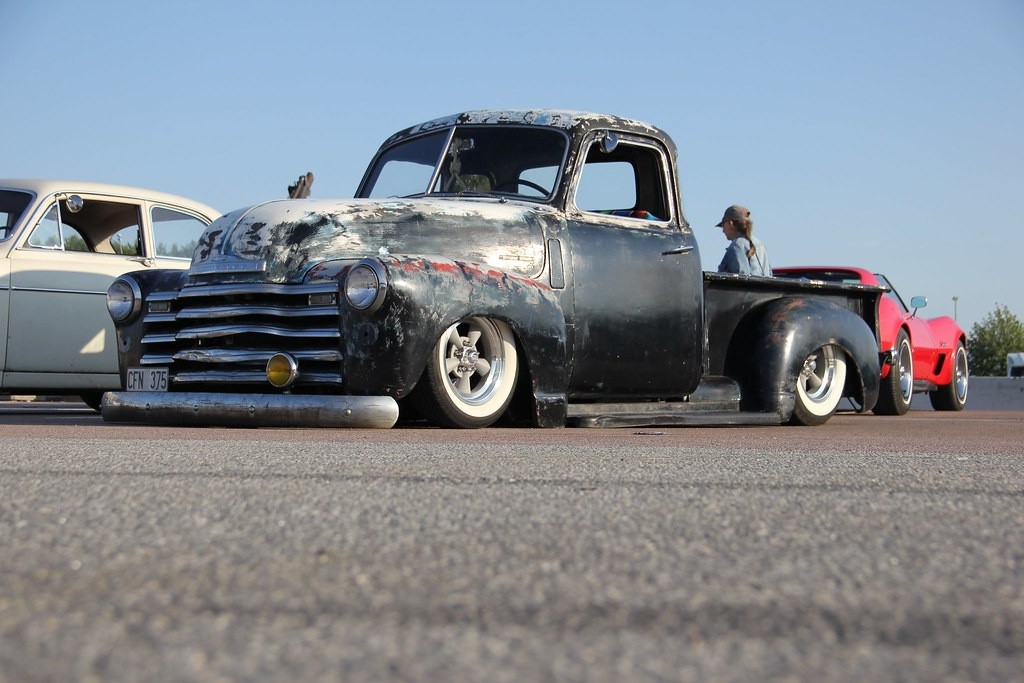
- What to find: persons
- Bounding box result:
[715,204,773,278]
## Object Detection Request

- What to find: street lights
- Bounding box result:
[953,297,959,320]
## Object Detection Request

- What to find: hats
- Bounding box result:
[714,205,750,227]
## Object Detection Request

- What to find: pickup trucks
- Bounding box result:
[101,107,891,441]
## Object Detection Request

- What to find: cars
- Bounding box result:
[772,267,968,416]
[0,179,229,410]
